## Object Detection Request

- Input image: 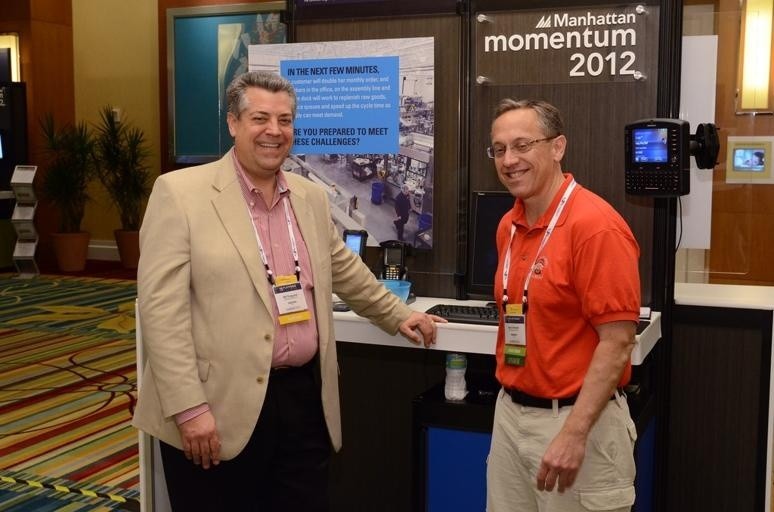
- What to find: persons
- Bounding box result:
[130,71,448,512]
[486,99,641,512]
[393,185,412,241]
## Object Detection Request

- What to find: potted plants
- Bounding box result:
[38,109,101,272]
[93,103,160,272]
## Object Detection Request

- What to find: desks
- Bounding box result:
[134,296,663,512]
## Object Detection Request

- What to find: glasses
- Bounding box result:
[487,135,558,159]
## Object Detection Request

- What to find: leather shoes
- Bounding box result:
[394,220,398,229]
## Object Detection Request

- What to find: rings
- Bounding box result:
[430,320,435,327]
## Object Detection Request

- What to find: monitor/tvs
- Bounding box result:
[467,191,515,296]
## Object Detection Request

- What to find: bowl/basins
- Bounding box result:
[377,279,411,304]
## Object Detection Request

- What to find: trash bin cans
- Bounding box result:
[417,214,432,232]
[371,183,383,204]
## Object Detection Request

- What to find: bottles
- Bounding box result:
[444,353,467,401]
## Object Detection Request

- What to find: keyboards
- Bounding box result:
[426,304,501,325]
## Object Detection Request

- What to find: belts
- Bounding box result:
[503,387,623,409]
